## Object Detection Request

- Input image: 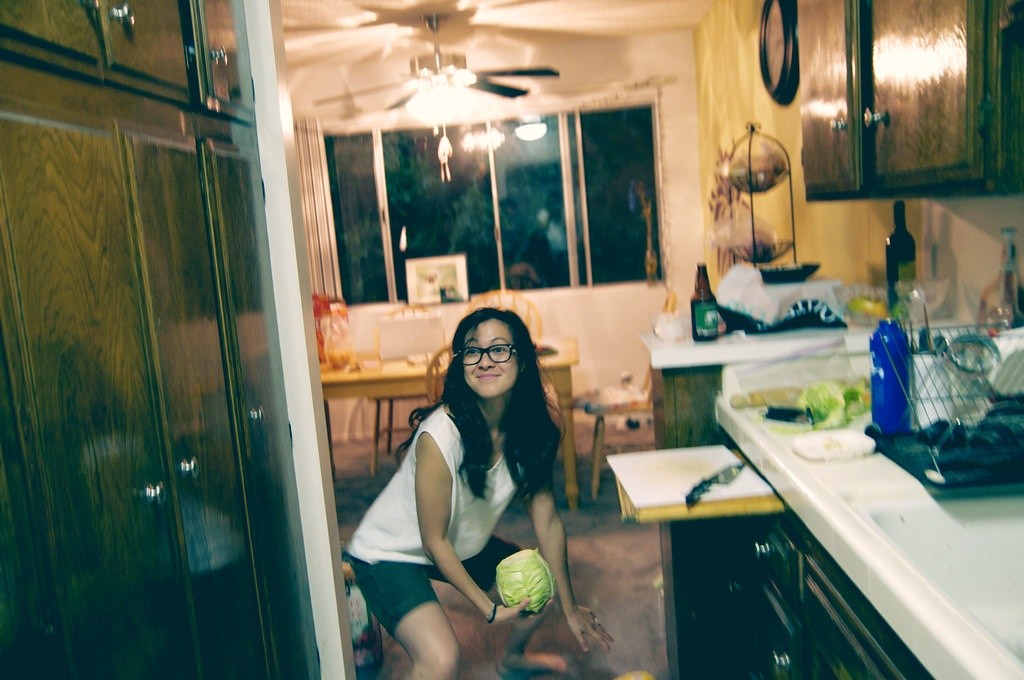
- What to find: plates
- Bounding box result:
[759,265,820,284]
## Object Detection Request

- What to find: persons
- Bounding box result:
[346,302,615,680]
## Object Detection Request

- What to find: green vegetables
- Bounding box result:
[799,381,873,430]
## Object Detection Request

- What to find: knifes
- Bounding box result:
[686,463,746,505]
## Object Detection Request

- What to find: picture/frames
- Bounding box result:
[405,254,470,303]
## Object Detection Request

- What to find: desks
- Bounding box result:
[321,342,578,511]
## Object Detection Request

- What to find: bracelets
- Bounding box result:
[487,604,497,624]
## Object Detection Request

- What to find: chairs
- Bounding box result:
[579,291,678,500]
[463,291,545,350]
[366,303,448,470]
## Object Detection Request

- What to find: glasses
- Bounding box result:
[459,344,516,366]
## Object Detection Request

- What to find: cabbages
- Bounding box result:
[496,546,556,612]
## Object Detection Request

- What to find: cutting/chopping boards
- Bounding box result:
[605,444,773,509]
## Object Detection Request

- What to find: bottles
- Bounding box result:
[870,320,911,438]
[986,226,1024,331]
[886,200,916,322]
[691,264,718,341]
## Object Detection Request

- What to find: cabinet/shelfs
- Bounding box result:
[0,0,323,680]
[800,2,1021,203]
[644,314,975,679]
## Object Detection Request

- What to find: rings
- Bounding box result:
[593,618,600,626]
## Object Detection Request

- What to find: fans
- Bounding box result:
[313,18,559,110]
